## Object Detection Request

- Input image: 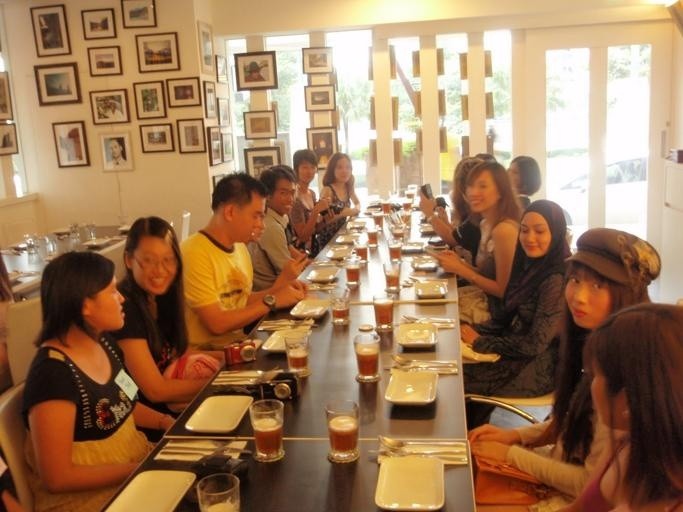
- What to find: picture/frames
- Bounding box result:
[134,31,182,74]
[196,17,237,192]
[132,80,167,119]
[120,0,158,29]
[301,44,339,173]
[139,122,176,153]
[88,88,131,125]
[78,7,118,41]
[99,132,135,174]
[29,4,73,59]
[165,77,203,108]
[175,117,208,155]
[361,44,498,169]
[31,61,83,107]
[232,50,286,181]
[51,120,91,168]
[86,46,124,77]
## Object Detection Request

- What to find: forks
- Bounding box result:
[157,449,232,462]
[367,449,466,464]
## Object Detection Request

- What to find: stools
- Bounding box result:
[461,390,555,423]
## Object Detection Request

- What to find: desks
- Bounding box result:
[97,194,479,512]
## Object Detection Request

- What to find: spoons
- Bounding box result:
[378,435,467,449]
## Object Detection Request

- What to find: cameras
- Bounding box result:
[436,196,447,208]
[320,201,344,216]
[260,374,300,402]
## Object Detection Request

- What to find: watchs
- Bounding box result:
[264,293,277,314]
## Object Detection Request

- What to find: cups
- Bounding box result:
[197,472,242,512]
[324,399,361,466]
[248,400,286,463]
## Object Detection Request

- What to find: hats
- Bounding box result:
[566,227,662,292]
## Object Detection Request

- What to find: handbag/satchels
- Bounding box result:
[473,443,563,505]
[167,353,220,413]
[461,334,502,364]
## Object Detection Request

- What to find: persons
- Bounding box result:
[468,226,660,511]
[317,152,360,251]
[460,200,572,427]
[178,171,305,351]
[21,251,175,511]
[248,150,330,291]
[108,216,226,415]
[106,139,127,169]
[562,303,683,511]
[420,151,542,325]
[98,99,123,119]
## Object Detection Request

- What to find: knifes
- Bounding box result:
[162,442,251,453]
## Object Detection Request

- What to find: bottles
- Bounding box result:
[0,219,98,273]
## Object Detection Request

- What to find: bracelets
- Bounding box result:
[159,414,170,428]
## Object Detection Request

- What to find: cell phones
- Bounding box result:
[424,245,438,254]
[421,183,433,199]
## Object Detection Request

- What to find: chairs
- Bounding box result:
[3,294,42,387]
[0,378,36,510]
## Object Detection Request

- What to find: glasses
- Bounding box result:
[135,255,177,269]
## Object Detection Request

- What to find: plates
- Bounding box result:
[374,455,445,511]
[182,183,456,434]
[101,468,197,512]
[80,236,111,251]
[116,225,132,231]
